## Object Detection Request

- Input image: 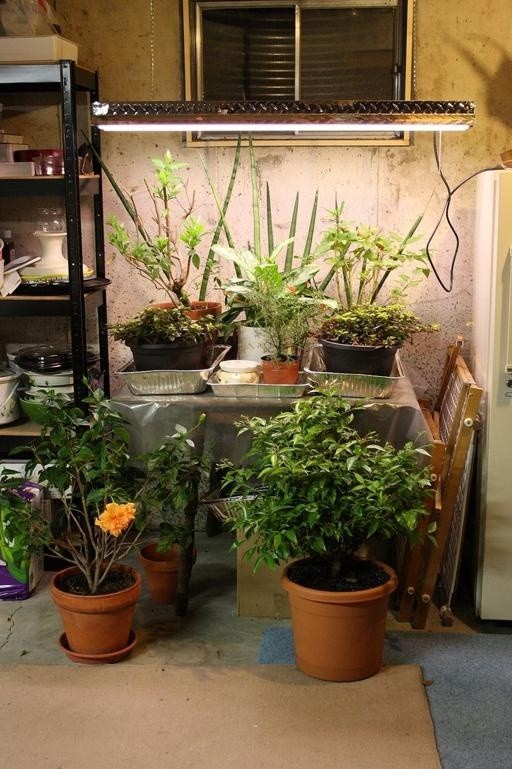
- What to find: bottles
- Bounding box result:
[2,230,16,264]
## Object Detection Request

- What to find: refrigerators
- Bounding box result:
[471,167,512,624]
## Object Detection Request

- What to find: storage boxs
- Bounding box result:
[233,518,370,620]
[0,36,79,63]
[1,459,73,499]
[0,161,35,176]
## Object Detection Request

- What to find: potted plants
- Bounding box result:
[104,304,222,369]
[215,381,440,682]
[196,131,353,332]
[106,150,222,368]
[210,237,339,366]
[248,287,313,385]
[314,300,440,377]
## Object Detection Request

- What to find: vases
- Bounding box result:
[19,231,89,277]
[135,538,192,603]
[37,205,68,234]
[49,560,142,654]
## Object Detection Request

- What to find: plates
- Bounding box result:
[218,359,259,373]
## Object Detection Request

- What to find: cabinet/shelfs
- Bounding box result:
[1,58,112,571]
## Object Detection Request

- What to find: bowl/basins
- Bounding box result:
[15,340,104,428]
[215,369,260,386]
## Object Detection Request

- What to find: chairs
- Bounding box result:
[396,340,483,630]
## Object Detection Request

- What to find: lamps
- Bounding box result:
[90,1,475,133]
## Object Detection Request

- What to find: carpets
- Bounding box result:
[252,622,512,769]
[1,664,443,769]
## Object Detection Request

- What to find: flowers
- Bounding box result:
[0,374,207,588]
